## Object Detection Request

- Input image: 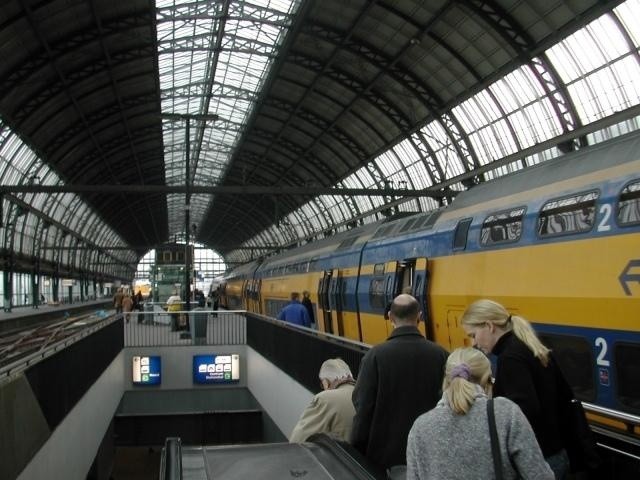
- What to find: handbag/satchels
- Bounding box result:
[571,399,598,445]
[178,316,187,326]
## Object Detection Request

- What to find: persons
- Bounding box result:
[402,344,559,480]
[286,358,361,446]
[111,283,221,333]
[302,290,317,336]
[352,293,457,480]
[275,291,311,328]
[457,297,608,480]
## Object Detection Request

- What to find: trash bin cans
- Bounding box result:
[143,304,154,325]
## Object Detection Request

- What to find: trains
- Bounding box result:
[212,129,640,461]
